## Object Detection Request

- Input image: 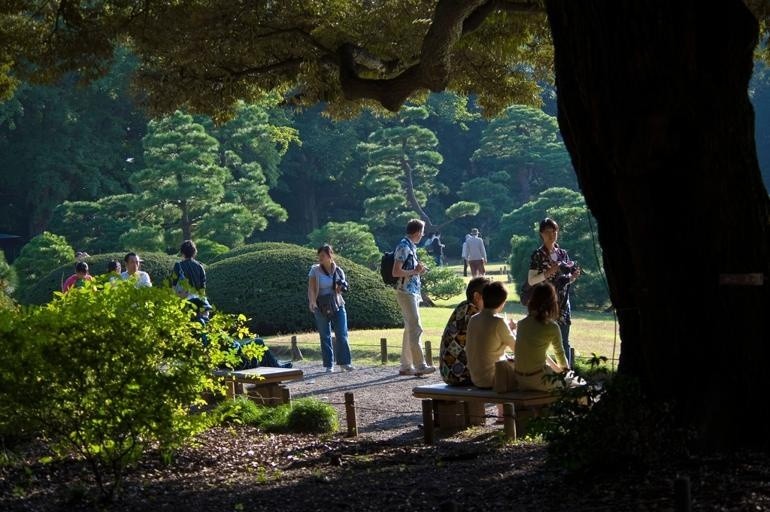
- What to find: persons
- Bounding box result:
[432,232,445,266]
[179,294,295,371]
[465,228,487,277]
[172,239,206,297]
[106,259,123,285]
[440,277,493,386]
[116,252,152,290]
[424,232,435,252]
[511,282,579,390]
[527,218,581,364]
[461,234,473,276]
[465,281,533,423]
[62,261,99,296]
[75,251,89,259]
[307,244,355,372]
[391,219,438,376]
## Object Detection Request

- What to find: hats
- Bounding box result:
[470,229,479,234]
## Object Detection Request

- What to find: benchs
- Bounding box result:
[411,383,594,447]
[216,367,306,407]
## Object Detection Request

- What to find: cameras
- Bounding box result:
[336,279,348,293]
[559,261,576,275]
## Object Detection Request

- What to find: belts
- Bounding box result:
[514,366,547,377]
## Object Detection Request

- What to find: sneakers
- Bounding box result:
[399,368,416,375]
[326,368,333,373]
[340,364,356,370]
[415,365,436,374]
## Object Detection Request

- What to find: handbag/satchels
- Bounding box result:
[520,279,537,306]
[381,241,414,286]
[174,274,190,297]
[318,294,340,316]
